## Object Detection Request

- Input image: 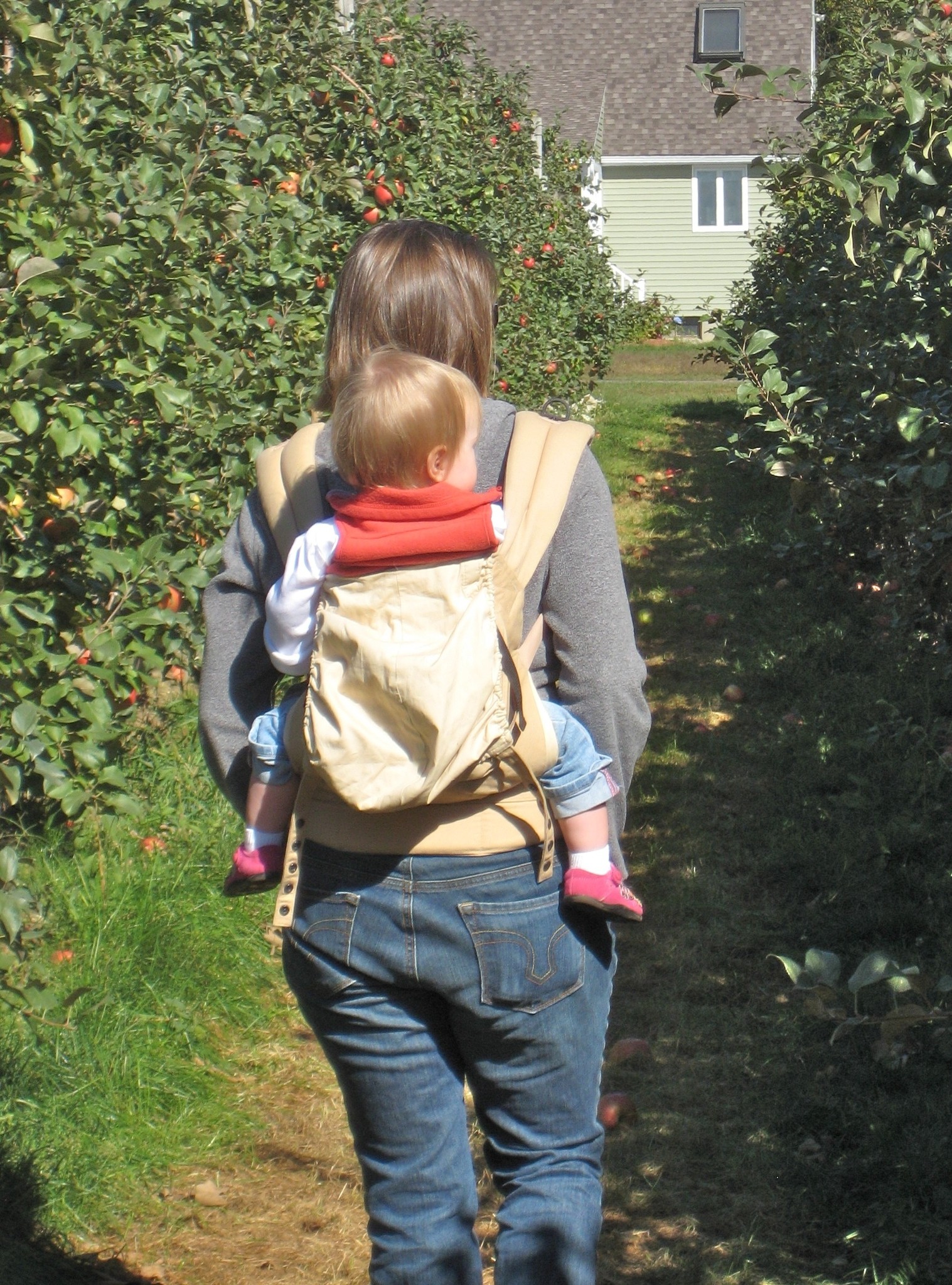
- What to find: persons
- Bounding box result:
[222,349,644,922]
[197,216,652,1285]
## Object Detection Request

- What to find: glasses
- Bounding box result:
[491,303,499,328]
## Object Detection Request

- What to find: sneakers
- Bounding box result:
[563,863,642,923]
[222,840,285,897]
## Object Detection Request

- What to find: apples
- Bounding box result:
[599,2,952,1127]
[0,0,555,961]
